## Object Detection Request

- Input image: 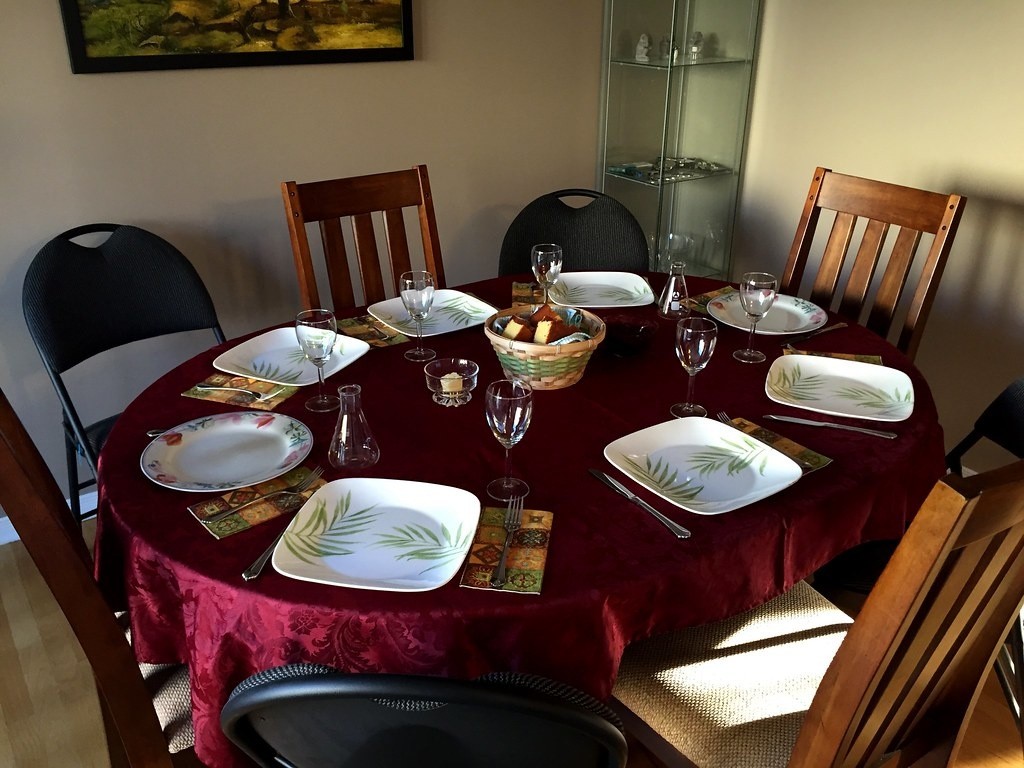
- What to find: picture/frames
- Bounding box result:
[57,0,414,74]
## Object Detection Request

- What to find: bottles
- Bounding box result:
[656,262,692,318]
[328,384,380,468]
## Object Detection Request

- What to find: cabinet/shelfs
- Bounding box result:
[596,0,762,281]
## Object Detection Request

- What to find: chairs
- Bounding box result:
[0,167,1023,768]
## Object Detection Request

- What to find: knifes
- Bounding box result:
[763,415,897,439]
[588,468,691,539]
[241,486,322,581]
[781,321,848,345]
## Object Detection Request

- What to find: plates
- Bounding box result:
[214,327,371,386]
[546,271,655,308]
[366,289,497,337]
[765,354,916,420]
[707,290,828,338]
[604,418,803,516]
[272,478,481,594]
[143,412,313,494]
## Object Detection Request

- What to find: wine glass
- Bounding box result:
[733,272,777,364]
[295,309,343,412]
[485,379,533,501]
[400,270,436,362]
[530,242,562,304]
[670,317,717,418]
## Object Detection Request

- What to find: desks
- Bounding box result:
[92,272,944,767]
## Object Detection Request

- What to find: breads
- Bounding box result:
[503,304,579,345]
[440,372,462,391]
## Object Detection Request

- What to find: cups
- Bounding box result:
[646,234,695,270]
[424,359,479,405]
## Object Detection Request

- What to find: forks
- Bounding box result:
[196,384,286,400]
[717,412,812,470]
[787,344,803,354]
[357,316,397,341]
[202,466,325,524]
[491,494,524,586]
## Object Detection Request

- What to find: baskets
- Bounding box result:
[484,304,606,391]
